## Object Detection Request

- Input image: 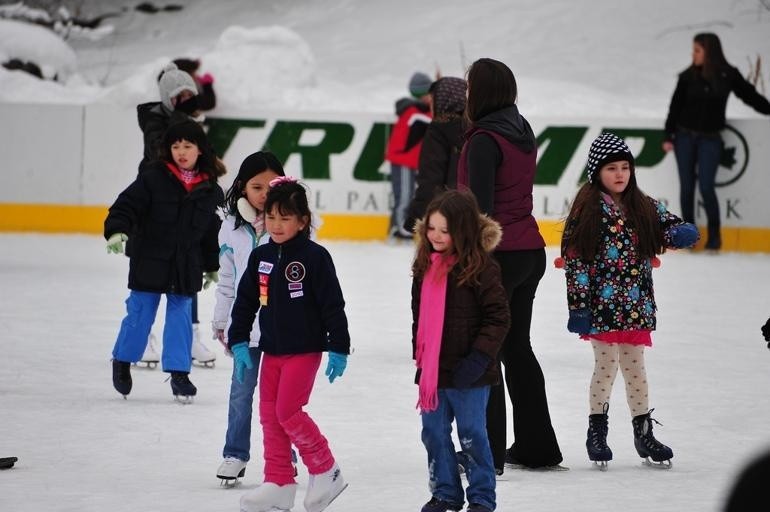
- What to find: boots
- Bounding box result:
[632,408,673,462]
[586,402,612,461]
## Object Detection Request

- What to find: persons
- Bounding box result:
[386,57,564,471]
[96,53,220,393]
[227,175,353,510]
[408,187,513,512]
[724,318,769,508]
[560,135,699,461]
[660,30,769,253]
[209,152,298,482]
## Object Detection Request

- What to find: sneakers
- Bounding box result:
[421,498,493,512]
[113,323,216,396]
[455,453,505,474]
[507,444,561,465]
[217,456,247,480]
[240,482,298,510]
[304,461,341,510]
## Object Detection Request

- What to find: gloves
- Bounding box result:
[568,308,593,335]
[669,225,699,249]
[107,233,129,254]
[231,341,254,385]
[203,270,218,290]
[325,350,347,384]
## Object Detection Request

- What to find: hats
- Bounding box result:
[410,73,432,96]
[586,133,635,186]
[159,62,205,123]
